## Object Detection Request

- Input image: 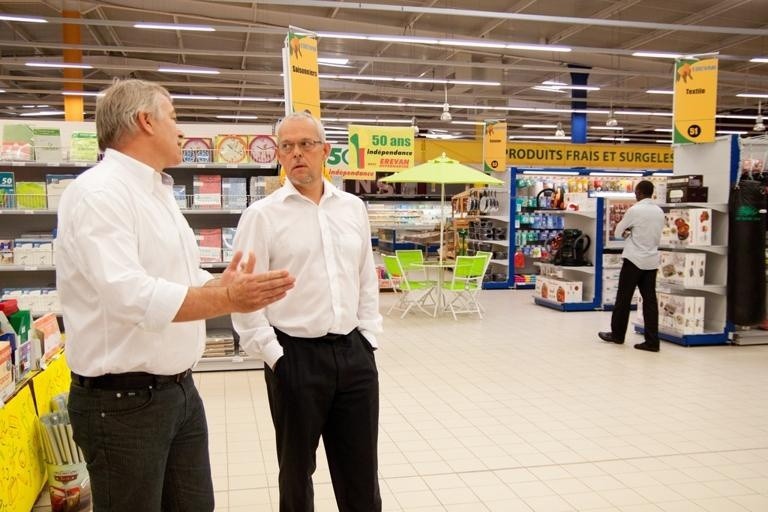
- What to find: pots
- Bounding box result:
[466,191,480,211]
[479,190,499,213]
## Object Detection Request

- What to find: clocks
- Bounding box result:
[177,136,213,164]
[212,132,249,165]
[247,133,278,164]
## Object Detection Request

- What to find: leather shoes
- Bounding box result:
[634,341,660,352]
[598,332,625,344]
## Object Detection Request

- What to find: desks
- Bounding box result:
[408,258,472,322]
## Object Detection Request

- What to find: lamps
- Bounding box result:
[605,91,619,127]
[439,82,456,122]
[752,100,767,133]
[552,114,569,137]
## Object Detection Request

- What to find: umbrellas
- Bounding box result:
[378,150,506,263]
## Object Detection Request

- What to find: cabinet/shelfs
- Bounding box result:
[448,161,587,290]
[628,138,741,345]
[0,124,291,374]
[529,190,654,313]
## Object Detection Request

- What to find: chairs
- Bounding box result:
[436,254,488,322]
[394,248,439,309]
[468,250,493,313]
[381,253,437,320]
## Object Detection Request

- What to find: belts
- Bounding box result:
[71,369,193,391]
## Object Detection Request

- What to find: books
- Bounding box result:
[222,176,248,210]
[191,227,223,264]
[192,173,222,210]
[222,226,238,263]
[250,175,282,204]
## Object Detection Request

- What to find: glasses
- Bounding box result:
[279,139,325,153]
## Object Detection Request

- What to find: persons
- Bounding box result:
[598,180,666,353]
[55,75,298,512]
[229,112,384,512]
